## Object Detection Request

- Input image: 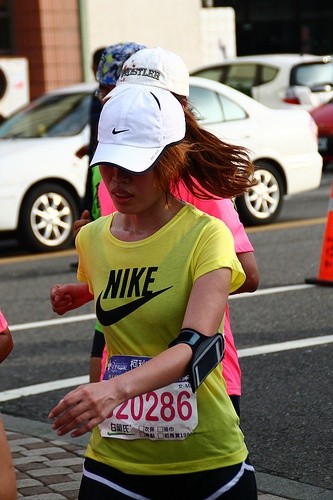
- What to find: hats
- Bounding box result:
[88,87,186,174]
[102,47,191,100]
[97,42,145,85]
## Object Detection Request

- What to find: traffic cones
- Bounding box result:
[305,189,333,287]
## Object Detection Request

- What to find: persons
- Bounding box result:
[0,309,14,364]
[46,84,258,500]
[49,47,259,418]
[68,41,147,381]
[0,416,18,500]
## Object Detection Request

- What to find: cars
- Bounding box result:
[187,52,333,162]
[0,74,326,251]
